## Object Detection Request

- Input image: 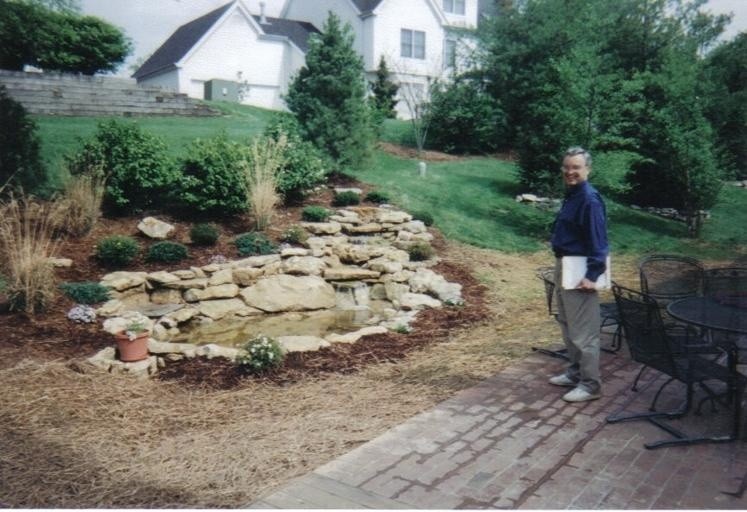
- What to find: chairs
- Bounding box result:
[562,387,600,402]
[549,374,577,386]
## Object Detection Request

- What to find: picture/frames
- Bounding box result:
[663,294,747,416]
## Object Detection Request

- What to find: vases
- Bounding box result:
[115,311,154,342]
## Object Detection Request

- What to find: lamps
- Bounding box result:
[531,266,627,364]
[630,252,739,415]
[604,282,743,454]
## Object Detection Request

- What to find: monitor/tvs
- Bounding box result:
[560,255,611,292]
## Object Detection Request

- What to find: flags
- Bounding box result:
[113,329,151,363]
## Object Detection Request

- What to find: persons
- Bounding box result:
[548,146,610,403]
